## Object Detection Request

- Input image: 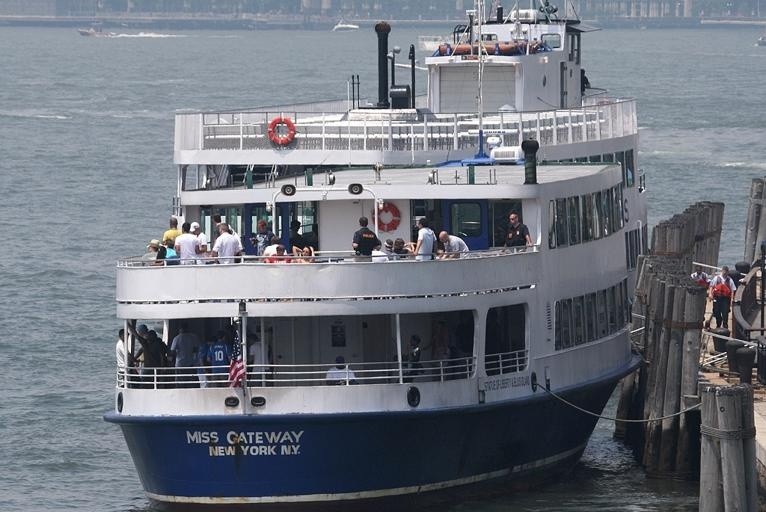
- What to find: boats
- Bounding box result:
[418,33,459,53]
[99,0,650,510]
[331,24,360,31]
[756,36,766,47]
[75,27,102,37]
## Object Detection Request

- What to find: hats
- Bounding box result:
[150,240,160,248]
[190,221,199,231]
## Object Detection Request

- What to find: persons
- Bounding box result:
[691,265,711,288]
[504,213,534,252]
[424,315,451,380]
[581,68,592,95]
[115,320,272,387]
[394,334,425,382]
[325,355,357,385]
[708,265,737,328]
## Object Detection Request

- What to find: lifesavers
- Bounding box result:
[371,202,399,232]
[267,117,294,145]
